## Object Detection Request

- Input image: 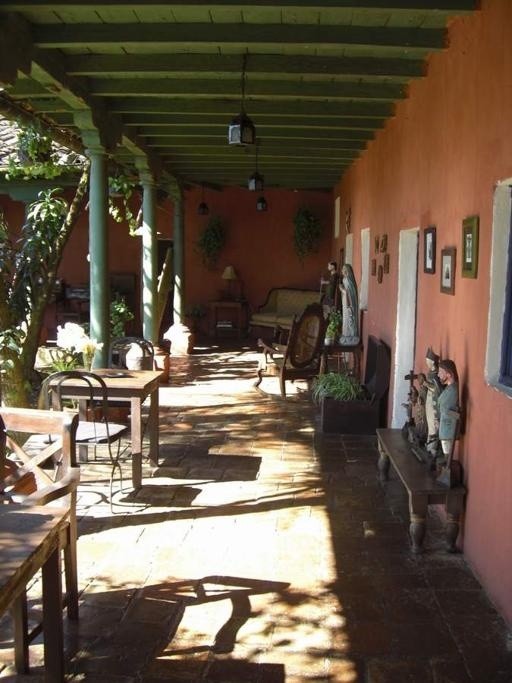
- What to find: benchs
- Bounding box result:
[370,418,465,561]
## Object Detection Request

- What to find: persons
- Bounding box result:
[437,359,461,470]
[320,260,339,317]
[340,262,358,337]
[418,349,442,451]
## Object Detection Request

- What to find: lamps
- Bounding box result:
[255,181,268,212]
[224,42,257,151]
[197,185,210,215]
[244,139,266,190]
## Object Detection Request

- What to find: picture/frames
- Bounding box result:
[460,213,480,281]
[440,243,457,296]
[421,225,437,276]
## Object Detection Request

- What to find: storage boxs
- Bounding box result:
[315,330,391,435]
